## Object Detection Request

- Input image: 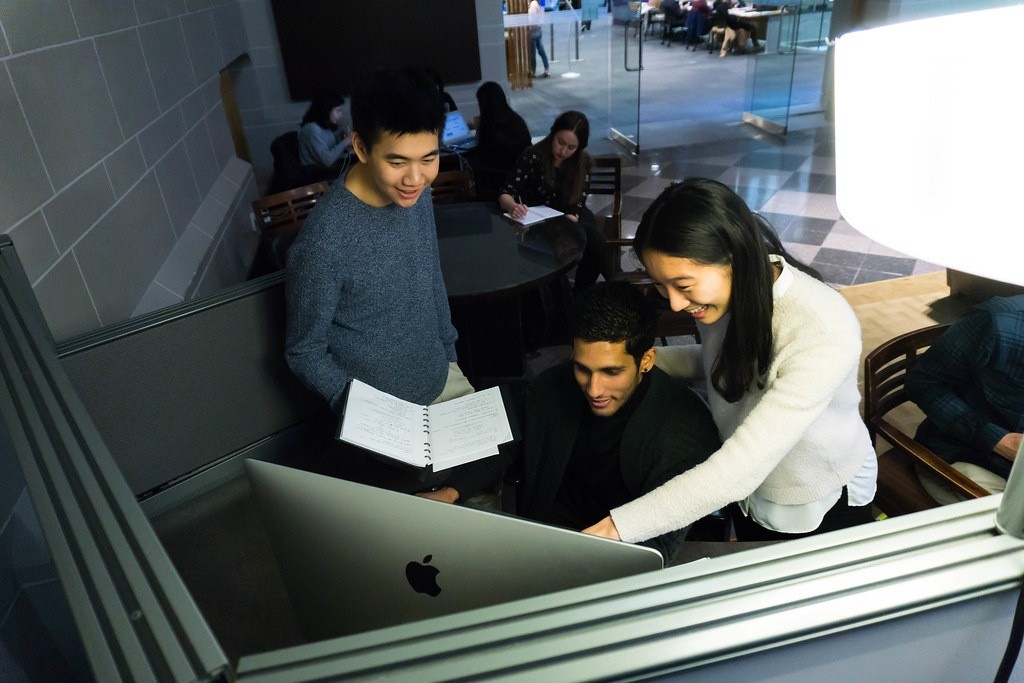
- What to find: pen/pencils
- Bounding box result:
[518,195,523,206]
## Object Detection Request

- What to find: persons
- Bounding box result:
[282,64,480,494]
[297,86,353,184]
[526,0,766,79]
[495,110,611,299]
[580,176,878,544]
[465,80,531,200]
[904,293,1024,505]
[416,278,723,565]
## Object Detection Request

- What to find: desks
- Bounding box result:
[149,472,784,659]
[729,6,789,40]
[434,203,587,376]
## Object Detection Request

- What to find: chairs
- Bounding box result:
[584,155,701,346]
[865,323,992,512]
[432,170,473,203]
[633,8,734,54]
[254,180,329,233]
[270,131,358,190]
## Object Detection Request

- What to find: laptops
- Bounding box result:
[440,109,479,150]
[245,458,664,643]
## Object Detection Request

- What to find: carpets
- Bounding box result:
[840,270,951,458]
